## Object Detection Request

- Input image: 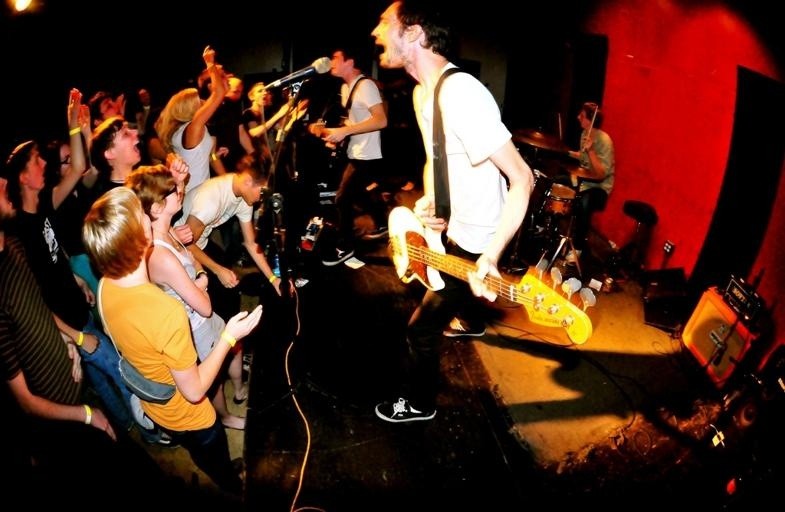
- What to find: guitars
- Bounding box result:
[388,207,597,346]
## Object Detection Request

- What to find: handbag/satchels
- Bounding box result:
[119,358,175,403]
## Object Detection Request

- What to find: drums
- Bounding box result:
[545,183,575,216]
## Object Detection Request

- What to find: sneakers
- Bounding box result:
[365,226,388,238]
[375,398,436,423]
[443,318,486,338]
[148,433,181,449]
[322,247,353,265]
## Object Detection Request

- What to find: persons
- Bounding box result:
[0,44,310,496]
[371,1,535,423]
[320,44,388,267]
[562,102,615,268]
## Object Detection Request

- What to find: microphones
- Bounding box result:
[267,55,334,92]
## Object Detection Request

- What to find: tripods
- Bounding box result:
[252,190,366,414]
[542,171,589,284]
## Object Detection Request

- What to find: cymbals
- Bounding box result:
[510,129,571,152]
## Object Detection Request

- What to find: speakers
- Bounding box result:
[643,268,691,333]
